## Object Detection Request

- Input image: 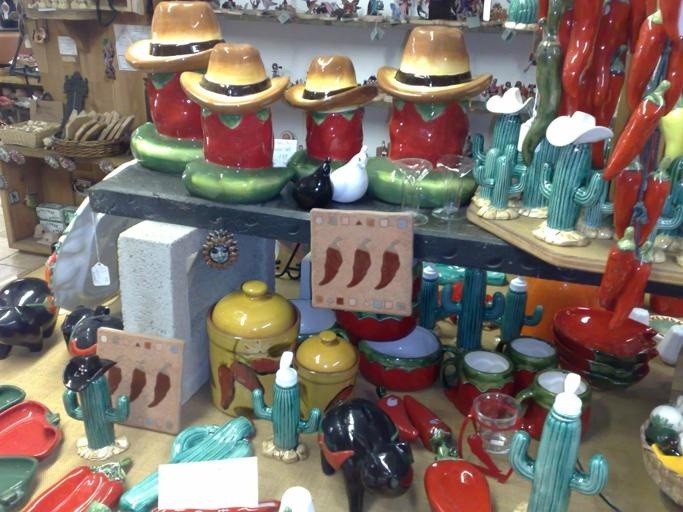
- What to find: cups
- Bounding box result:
[353,326,593,442]
[471,392,522,456]
[392,154,477,227]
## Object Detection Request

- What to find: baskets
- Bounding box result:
[52,124,130,158]
[639,398,683,506]
[0,119,61,147]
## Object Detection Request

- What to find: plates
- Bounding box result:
[0,383,63,512]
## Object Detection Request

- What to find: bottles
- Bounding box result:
[206,280,361,423]
